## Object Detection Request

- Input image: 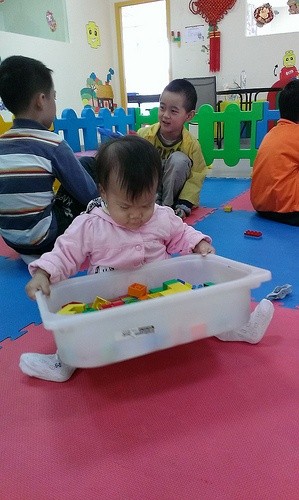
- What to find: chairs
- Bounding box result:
[183,76,222,149]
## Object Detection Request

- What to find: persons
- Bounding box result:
[18,136,274,381]
[250,79,299,226]
[136,80,207,218]
[0,56,100,256]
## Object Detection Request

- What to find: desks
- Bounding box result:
[216,88,283,148]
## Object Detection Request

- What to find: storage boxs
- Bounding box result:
[34,253,271,369]
